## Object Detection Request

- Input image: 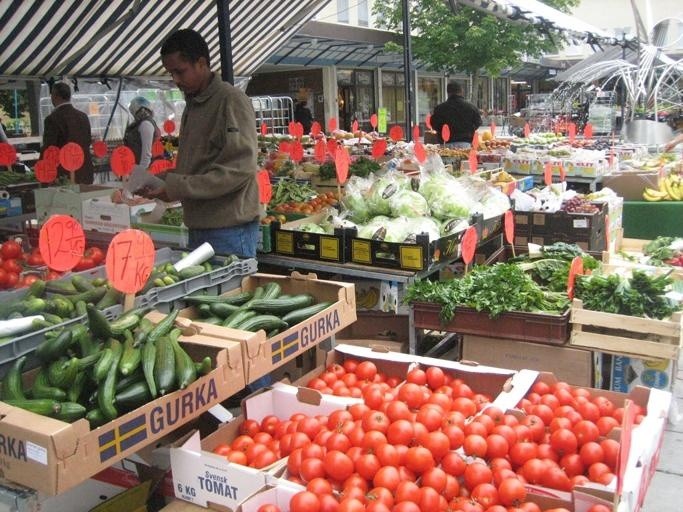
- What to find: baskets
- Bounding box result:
[0,246,259,383]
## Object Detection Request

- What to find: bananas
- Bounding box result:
[357,286,379,309]
[641,174,683,202]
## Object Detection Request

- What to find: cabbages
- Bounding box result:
[299,177,510,243]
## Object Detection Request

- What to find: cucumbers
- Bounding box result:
[0,252,336,430]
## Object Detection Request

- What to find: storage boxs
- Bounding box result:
[460,331,592,392]
[590,337,676,404]
[132,219,188,251]
[79,191,183,231]
[350,310,426,351]
[603,168,670,200]
[339,273,384,311]
[188,148,609,265]
[412,280,570,346]
[33,183,118,234]
[141,270,359,386]
[0,322,245,493]
[168,341,673,512]
[377,277,412,318]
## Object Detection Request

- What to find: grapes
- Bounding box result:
[559,196,600,213]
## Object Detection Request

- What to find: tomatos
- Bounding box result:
[214,358,646,512]
[262,192,339,224]
[0,240,107,290]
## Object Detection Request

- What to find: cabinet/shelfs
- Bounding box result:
[568,291,680,360]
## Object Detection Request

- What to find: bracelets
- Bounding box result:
[670,140,676,146]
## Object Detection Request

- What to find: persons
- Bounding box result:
[665,133,683,151]
[294,101,314,135]
[430,82,483,144]
[134,29,272,409]
[122,96,161,168]
[39,80,94,185]
